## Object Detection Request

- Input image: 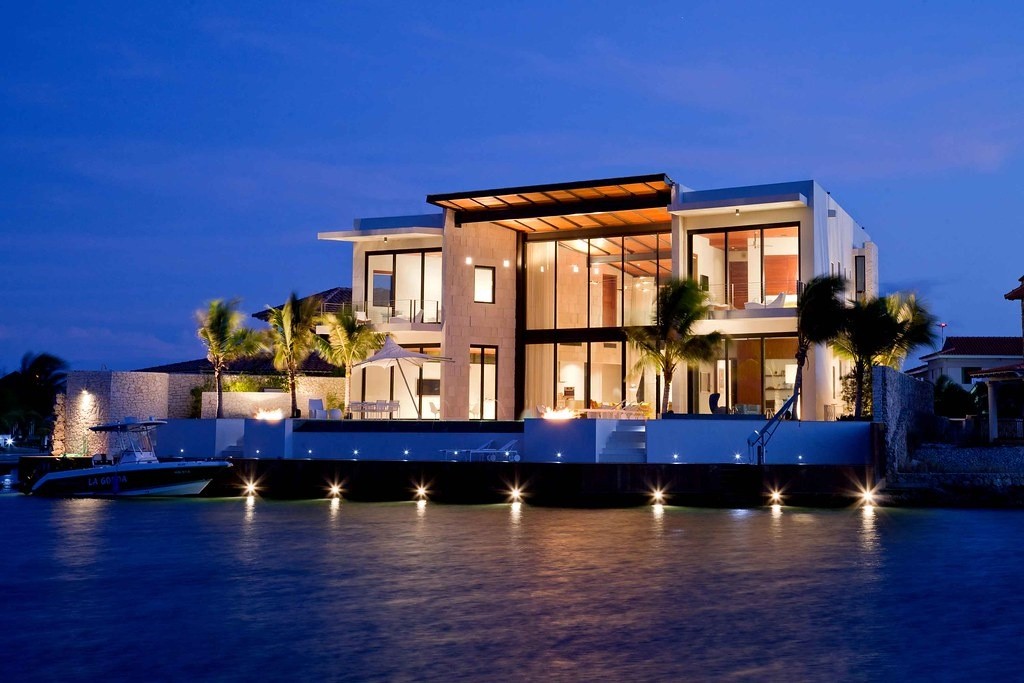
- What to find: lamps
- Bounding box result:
[735,209,739,216]
[384,237,387,243]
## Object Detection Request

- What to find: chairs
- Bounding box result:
[534,399,644,420]
[437,437,520,462]
[347,398,400,419]
[708,391,731,414]
[308,398,327,420]
[389,308,424,323]
[429,401,440,419]
[92,453,114,468]
[742,291,786,308]
[355,311,371,325]
[734,403,760,414]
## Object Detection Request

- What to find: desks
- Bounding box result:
[350,402,400,420]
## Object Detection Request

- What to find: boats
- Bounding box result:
[28,419,233,499]
